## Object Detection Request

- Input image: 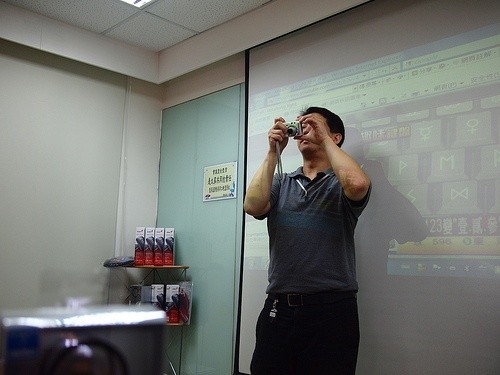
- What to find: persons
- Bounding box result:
[243,104,372,375]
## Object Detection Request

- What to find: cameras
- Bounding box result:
[280,120,303,137]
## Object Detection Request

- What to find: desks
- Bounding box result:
[108,265,189,375]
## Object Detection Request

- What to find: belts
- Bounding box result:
[269,292,355,308]
